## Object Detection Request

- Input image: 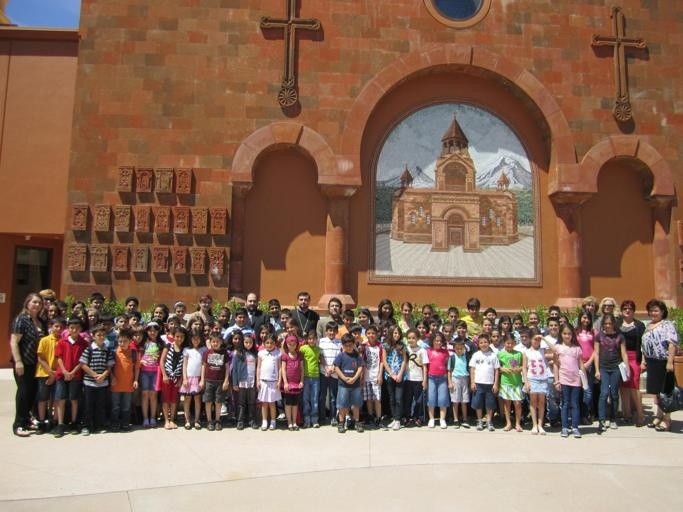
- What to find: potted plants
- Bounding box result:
[667,305,682,390]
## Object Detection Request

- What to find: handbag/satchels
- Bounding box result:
[658,387,682,413]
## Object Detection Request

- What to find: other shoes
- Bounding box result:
[13,413,671,436]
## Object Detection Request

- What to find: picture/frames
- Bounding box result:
[363,94,545,290]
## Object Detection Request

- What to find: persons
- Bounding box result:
[11,289,679,437]
